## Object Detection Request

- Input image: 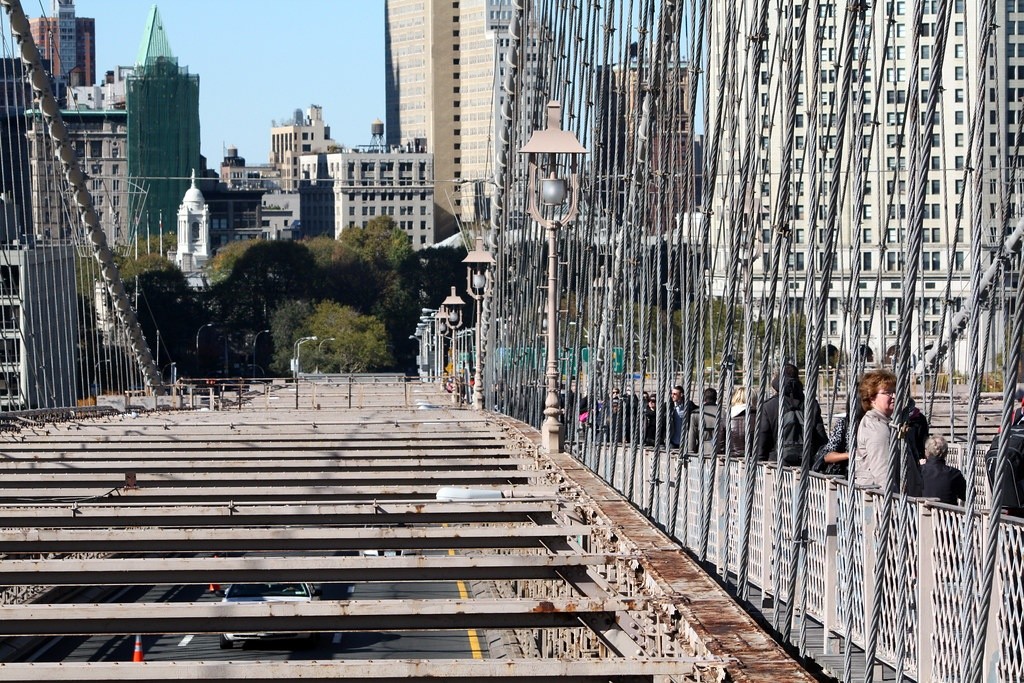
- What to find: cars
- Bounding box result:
[215,582,320,648]
[358,524,420,557]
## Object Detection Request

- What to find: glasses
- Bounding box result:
[876,391,896,396]
[648,399,655,403]
[671,393,681,396]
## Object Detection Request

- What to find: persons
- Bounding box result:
[447,365,828,468]
[997,375,1024,680]
[823,373,975,506]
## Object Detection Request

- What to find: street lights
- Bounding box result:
[294,336,317,378]
[433,305,450,373]
[196,324,212,349]
[319,338,335,351]
[409,308,444,383]
[253,330,269,377]
[442,286,466,393]
[517,101,590,452]
[461,236,497,410]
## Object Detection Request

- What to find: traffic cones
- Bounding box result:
[133,634,144,662]
[210,556,220,591]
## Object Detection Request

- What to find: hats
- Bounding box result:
[772,365,803,394]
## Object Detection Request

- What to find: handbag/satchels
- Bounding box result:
[579,412,589,422]
[812,418,847,475]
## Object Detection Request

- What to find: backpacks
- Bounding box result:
[985,409,1024,510]
[776,394,804,466]
